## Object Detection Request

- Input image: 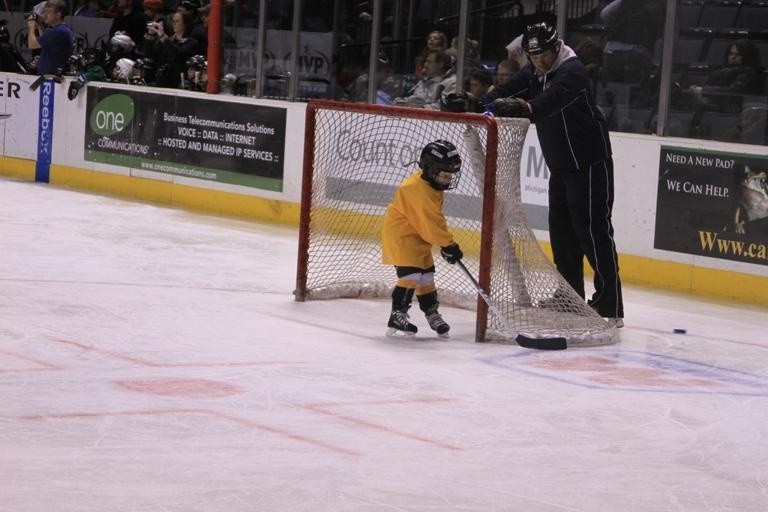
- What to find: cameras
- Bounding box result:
[24,11,43,25]
[145,21,159,30]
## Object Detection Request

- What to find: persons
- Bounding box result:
[379,139,463,338]
[328,1,617,114]
[446,19,625,329]
[0,0,237,94]
[670,36,763,111]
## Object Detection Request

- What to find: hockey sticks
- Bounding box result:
[456,261,567,350]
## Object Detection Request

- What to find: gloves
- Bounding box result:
[53,67,64,84]
[447,91,489,112]
[67,81,82,101]
[440,242,463,264]
[494,97,531,118]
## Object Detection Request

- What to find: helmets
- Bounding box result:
[66,48,94,73]
[186,54,209,75]
[113,58,134,81]
[110,34,137,58]
[521,22,561,56]
[133,58,158,83]
[419,139,462,191]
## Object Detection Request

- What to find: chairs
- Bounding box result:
[574,1,768,146]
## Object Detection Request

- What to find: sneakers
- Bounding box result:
[388,305,418,334]
[424,301,450,334]
[537,290,585,313]
[578,302,624,318]
